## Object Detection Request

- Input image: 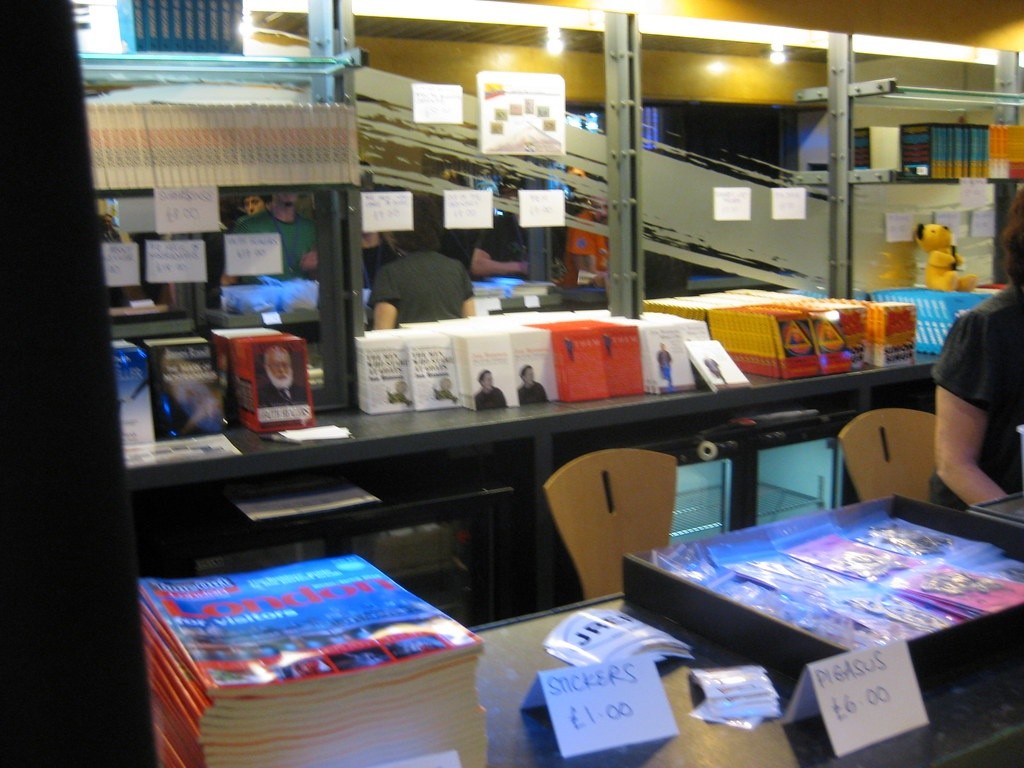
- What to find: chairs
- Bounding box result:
[543,447,677,600]
[838,408,936,501]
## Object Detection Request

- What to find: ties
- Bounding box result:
[279,389,290,401]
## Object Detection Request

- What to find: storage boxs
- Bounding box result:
[621,491,1024,692]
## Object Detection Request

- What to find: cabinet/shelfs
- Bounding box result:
[794,75,1024,186]
[126,352,939,628]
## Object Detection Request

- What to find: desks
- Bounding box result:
[469,592,1024,768]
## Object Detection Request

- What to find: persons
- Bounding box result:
[701,355,727,387]
[360,166,611,332]
[927,185,1024,514]
[475,368,506,410]
[258,344,307,409]
[517,363,549,406]
[656,341,674,388]
[96,196,136,243]
[220,193,320,284]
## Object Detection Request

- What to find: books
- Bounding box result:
[898,123,1024,179]
[684,275,778,290]
[641,288,916,380]
[353,308,752,411]
[110,327,316,446]
[81,83,361,191]
[220,280,323,318]
[853,126,902,172]
[116,0,243,55]
[139,551,490,768]
[870,288,997,357]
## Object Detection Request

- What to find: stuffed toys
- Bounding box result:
[880,240,918,286]
[914,222,976,292]
